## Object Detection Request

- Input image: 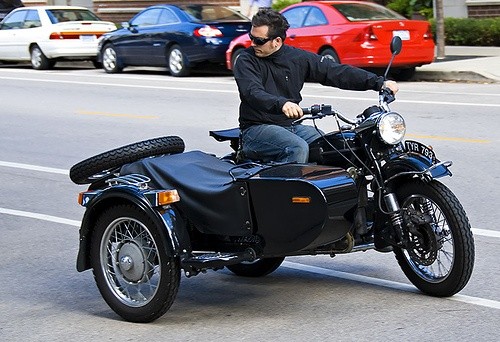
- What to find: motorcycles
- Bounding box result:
[70,35,474,323]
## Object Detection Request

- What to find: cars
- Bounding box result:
[225,0,434,80]
[98,2,255,78]
[0,5,117,70]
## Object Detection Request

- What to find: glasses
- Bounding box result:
[248,33,274,45]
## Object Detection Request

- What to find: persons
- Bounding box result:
[233,7,400,165]
